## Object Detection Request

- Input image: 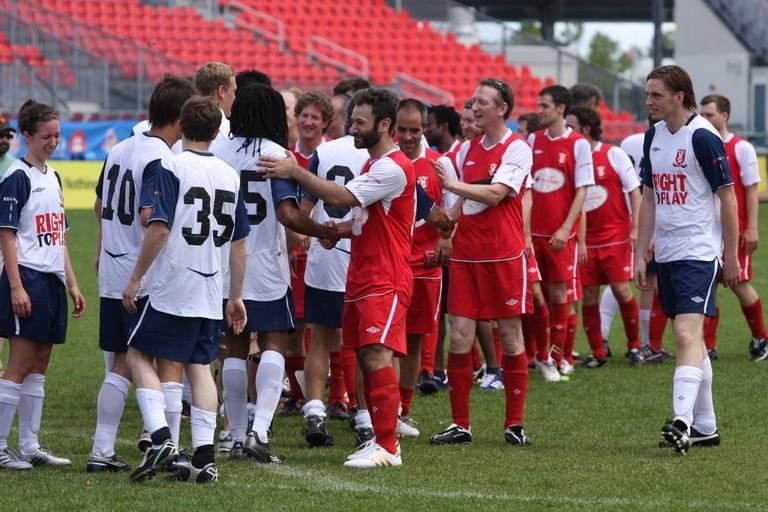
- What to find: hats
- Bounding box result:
[0,125,17,133]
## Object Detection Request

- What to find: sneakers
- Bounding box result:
[661,419,690,456]
[440,365,529,393]
[429,423,472,445]
[355,427,376,446]
[504,424,532,446]
[16,447,72,467]
[130,399,278,483]
[0,447,34,470]
[534,341,663,383]
[342,436,403,470]
[417,367,439,396]
[394,417,421,439]
[749,331,768,363]
[245,378,333,447]
[327,401,358,429]
[658,425,720,448]
[86,453,133,473]
[709,347,719,364]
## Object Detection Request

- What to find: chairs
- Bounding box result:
[0,0,647,145]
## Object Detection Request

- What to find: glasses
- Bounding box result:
[0,134,13,140]
